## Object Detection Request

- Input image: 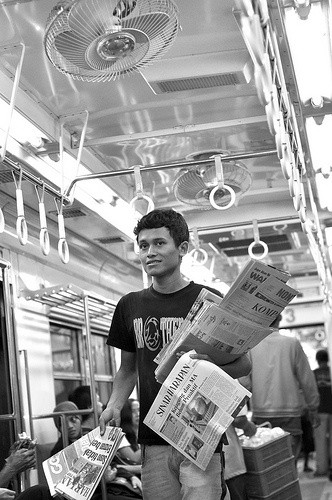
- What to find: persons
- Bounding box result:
[101,208,250,500]
[102,400,143,462]
[0,448,62,500]
[123,399,141,451]
[311,349,332,477]
[217,414,261,500]
[234,311,318,469]
[50,386,143,500]
[294,412,313,472]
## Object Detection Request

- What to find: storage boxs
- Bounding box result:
[231,422,304,500]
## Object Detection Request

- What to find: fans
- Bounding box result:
[172,150,252,210]
[42,0,180,84]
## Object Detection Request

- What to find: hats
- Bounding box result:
[53,401,82,429]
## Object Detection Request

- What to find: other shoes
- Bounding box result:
[303,467,313,472]
